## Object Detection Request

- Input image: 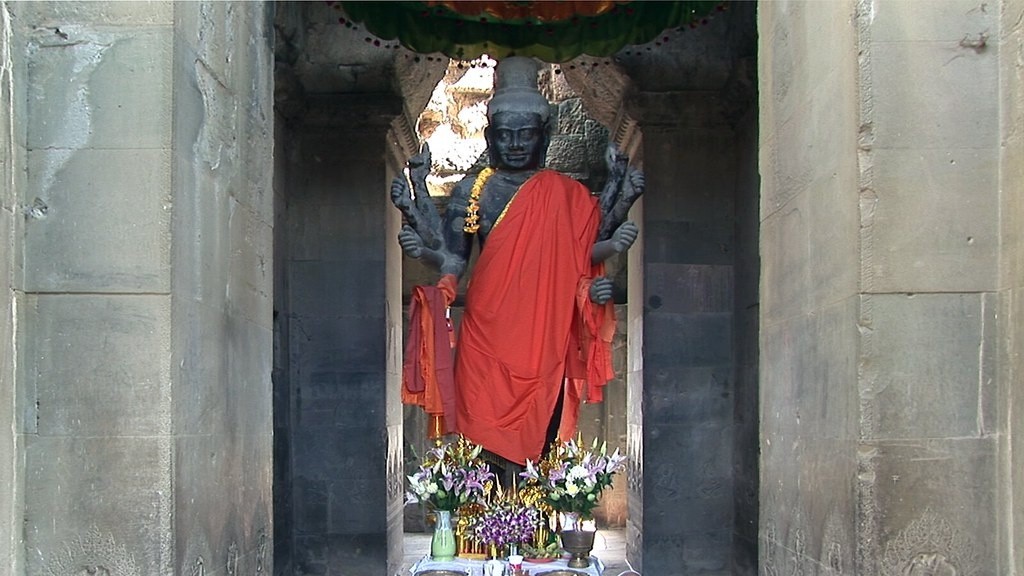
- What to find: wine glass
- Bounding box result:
[560,530,597,568]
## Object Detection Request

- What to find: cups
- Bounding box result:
[483,560,504,576]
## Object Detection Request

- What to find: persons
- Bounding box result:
[390,58,650,492]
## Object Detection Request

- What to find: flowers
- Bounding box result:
[403,438,628,544]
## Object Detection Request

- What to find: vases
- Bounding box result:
[430,510,455,561]
[504,542,518,576]
[560,512,581,557]
